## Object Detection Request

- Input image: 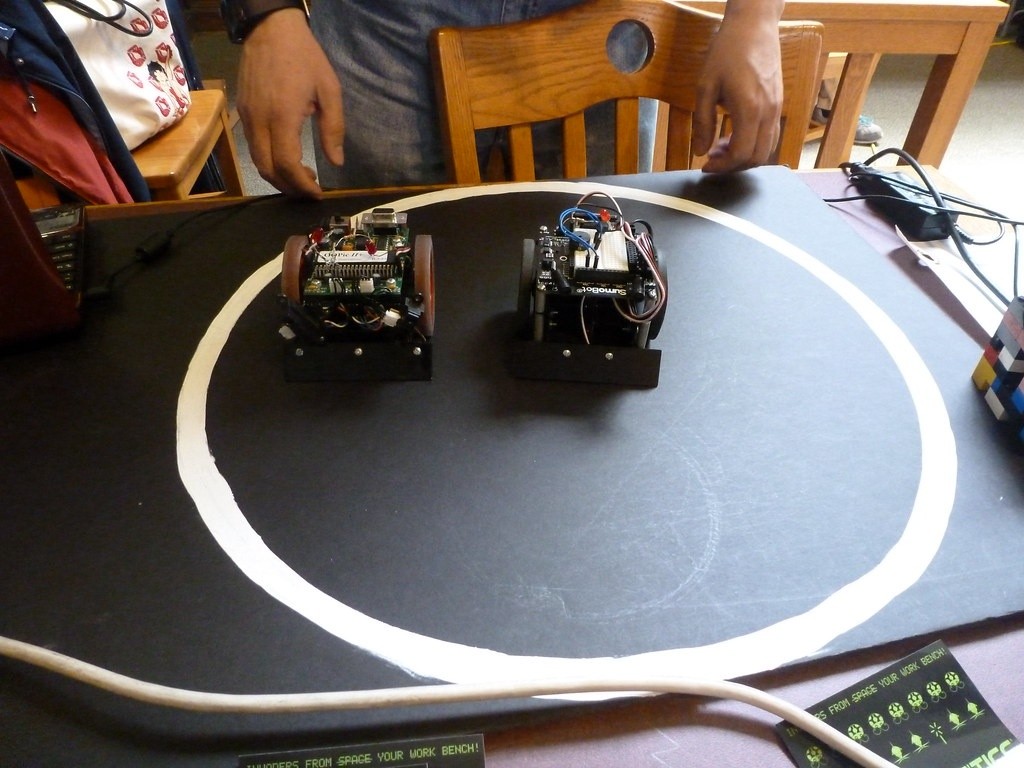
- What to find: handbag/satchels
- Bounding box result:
[43,1,193,154]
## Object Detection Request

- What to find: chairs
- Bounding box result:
[429,0,826,185]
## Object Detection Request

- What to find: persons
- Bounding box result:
[810,79,882,144]
[221,0,784,198]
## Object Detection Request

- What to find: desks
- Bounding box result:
[652,0,1010,171]
[0,164,1024,767]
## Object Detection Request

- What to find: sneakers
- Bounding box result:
[813,107,884,145]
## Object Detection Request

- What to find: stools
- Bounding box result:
[127,91,247,200]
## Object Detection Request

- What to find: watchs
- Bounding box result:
[225,0,309,44]
